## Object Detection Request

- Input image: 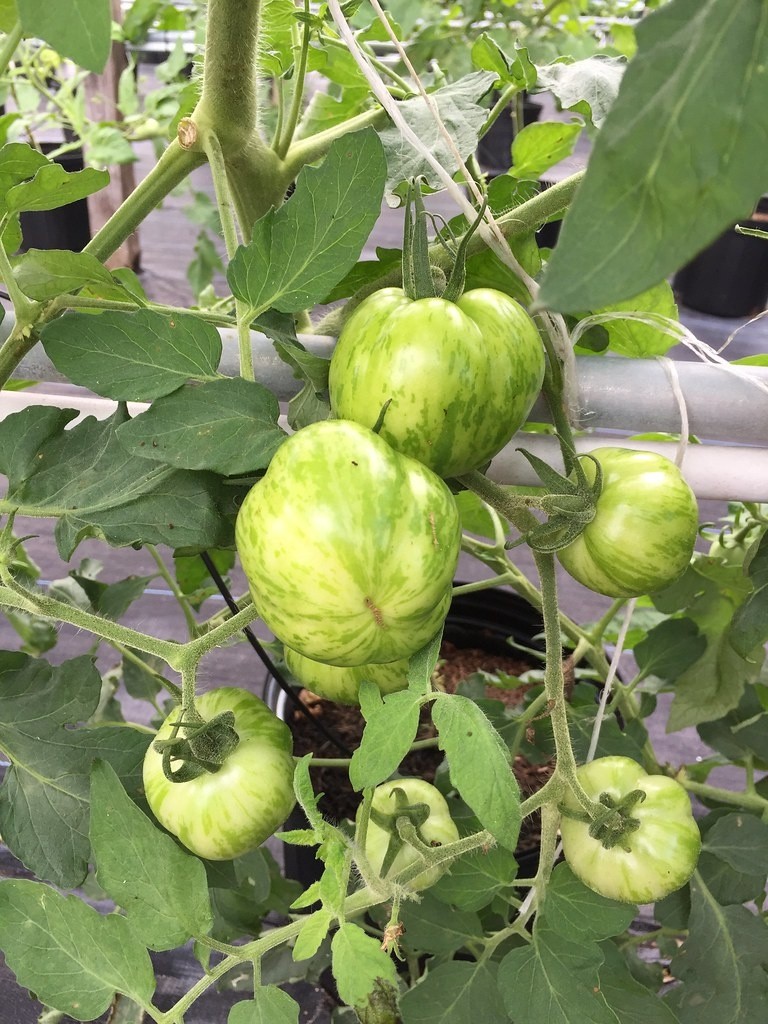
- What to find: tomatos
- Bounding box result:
[125,287,699,902]
